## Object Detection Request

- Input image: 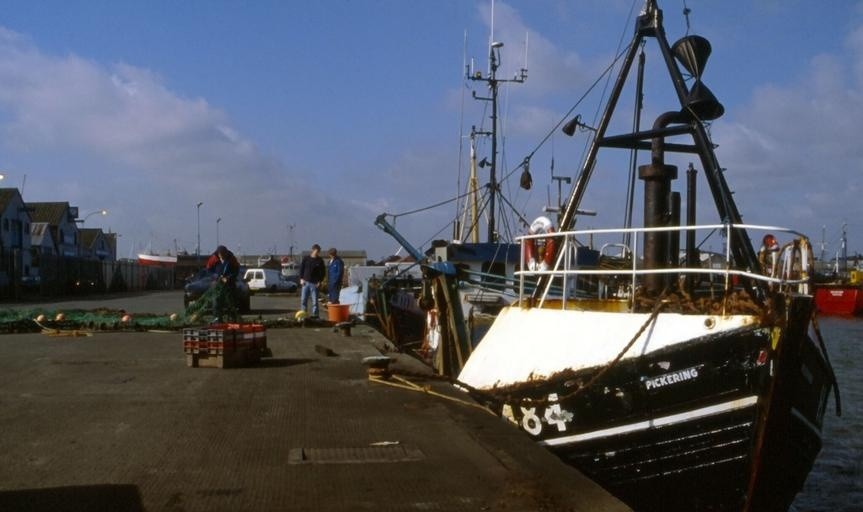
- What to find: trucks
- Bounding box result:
[243,268,298,293]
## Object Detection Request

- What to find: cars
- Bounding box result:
[72,276,97,293]
[21,266,40,288]
[180,268,252,312]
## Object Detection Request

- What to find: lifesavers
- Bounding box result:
[524,216,554,271]
[756,235,776,290]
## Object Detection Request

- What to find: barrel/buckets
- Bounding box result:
[327,304,349,322]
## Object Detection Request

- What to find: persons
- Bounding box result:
[298,243,326,317]
[206,245,241,324]
[327,248,344,304]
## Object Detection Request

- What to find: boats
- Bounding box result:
[369,0,824,337]
[440,0,846,512]
[812,221,861,318]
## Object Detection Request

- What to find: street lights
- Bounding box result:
[215,217,221,246]
[195,200,203,267]
[83,210,107,220]
